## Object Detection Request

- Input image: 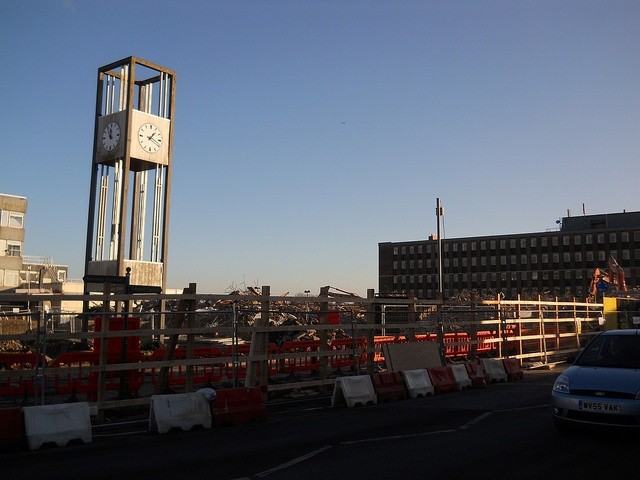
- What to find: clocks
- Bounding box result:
[138,122,163,154]
[102,121,121,152]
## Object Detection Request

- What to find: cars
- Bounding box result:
[552,329,639,429]
